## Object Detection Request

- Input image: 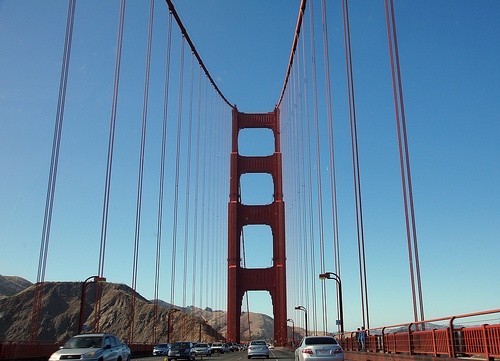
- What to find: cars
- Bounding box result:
[205,341,249,354]
[48,333,132,361]
[152,343,169,356]
[166,341,196,361]
[192,342,212,357]
[294,335,345,361]
[248,340,270,359]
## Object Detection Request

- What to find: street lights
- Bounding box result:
[77,275,107,334]
[199,320,207,342]
[318,271,344,350]
[287,318,295,347]
[295,306,307,337]
[167,308,181,343]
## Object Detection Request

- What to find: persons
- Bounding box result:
[356,327,368,351]
